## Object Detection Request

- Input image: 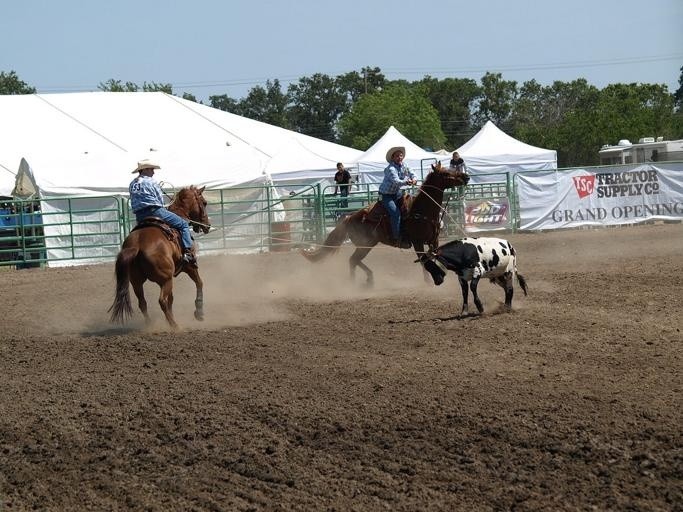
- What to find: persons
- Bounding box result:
[332,162,351,216]
[375,145,418,246]
[127,157,194,263]
[447,151,469,198]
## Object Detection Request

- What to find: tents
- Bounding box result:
[445,120,558,183]
[354,126,445,191]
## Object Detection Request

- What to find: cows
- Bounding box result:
[411,235,529,314]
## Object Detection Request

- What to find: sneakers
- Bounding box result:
[394,241,410,248]
[184,249,198,269]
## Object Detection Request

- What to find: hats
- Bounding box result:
[132,159,161,173]
[386,146,406,162]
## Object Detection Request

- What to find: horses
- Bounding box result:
[104,184,211,332]
[300,159,470,287]
